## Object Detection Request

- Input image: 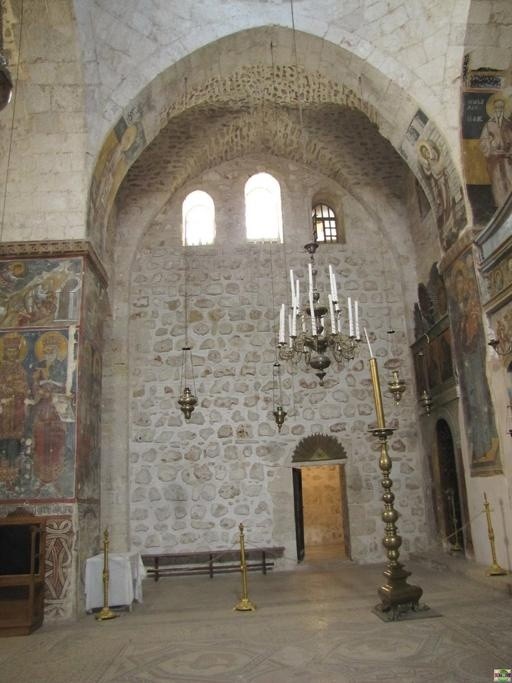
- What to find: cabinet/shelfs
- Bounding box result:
[1,519,47,637]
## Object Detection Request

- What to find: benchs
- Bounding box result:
[142,546,285,582]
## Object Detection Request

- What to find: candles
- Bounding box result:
[278,264,361,347]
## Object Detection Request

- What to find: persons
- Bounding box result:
[478,98,512,208]
[0,262,72,492]
[449,270,502,467]
